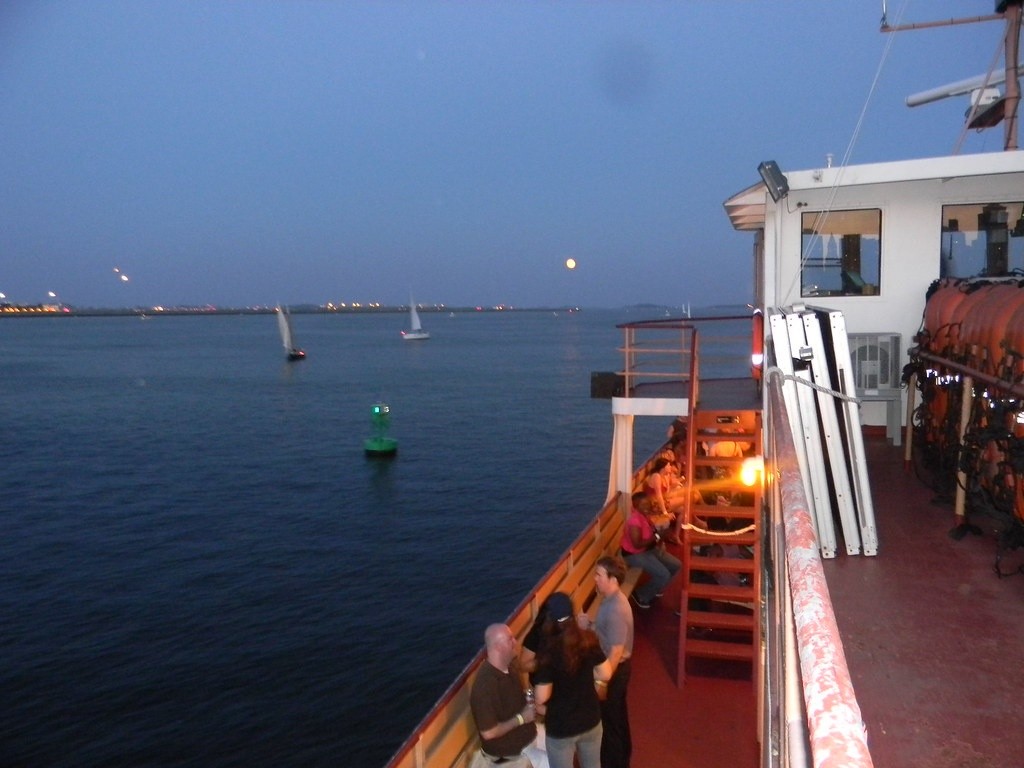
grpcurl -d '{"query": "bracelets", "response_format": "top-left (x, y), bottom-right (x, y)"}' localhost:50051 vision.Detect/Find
top-left (515, 712), bottom-right (525, 725)
top-left (595, 680), bottom-right (607, 687)
top-left (655, 533), bottom-right (660, 539)
top-left (664, 512), bottom-right (667, 515)
top-left (587, 620), bottom-right (592, 630)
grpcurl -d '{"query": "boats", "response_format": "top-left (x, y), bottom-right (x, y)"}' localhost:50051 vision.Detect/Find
top-left (364, 400), bottom-right (398, 455)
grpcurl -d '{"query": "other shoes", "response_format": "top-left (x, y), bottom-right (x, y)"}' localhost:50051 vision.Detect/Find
top-left (656, 591), bottom-right (664, 597)
top-left (631, 591), bottom-right (651, 609)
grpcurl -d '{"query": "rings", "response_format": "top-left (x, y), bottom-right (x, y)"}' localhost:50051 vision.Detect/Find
top-left (673, 519), bottom-right (674, 520)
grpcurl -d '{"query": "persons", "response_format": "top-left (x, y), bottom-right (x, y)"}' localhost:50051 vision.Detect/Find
top-left (469, 622), bottom-right (552, 768)
top-left (532, 592), bottom-right (614, 768)
top-left (575, 555), bottom-right (634, 768)
top-left (620, 416), bottom-right (755, 615)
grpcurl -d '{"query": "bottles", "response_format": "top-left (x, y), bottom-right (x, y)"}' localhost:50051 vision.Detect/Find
top-left (525, 689), bottom-right (535, 708)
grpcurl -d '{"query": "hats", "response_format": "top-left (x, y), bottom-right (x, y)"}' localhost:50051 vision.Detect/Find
top-left (546, 592), bottom-right (572, 623)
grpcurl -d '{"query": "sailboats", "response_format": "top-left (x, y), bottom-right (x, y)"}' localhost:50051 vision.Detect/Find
top-left (399, 292), bottom-right (431, 339)
top-left (275, 303), bottom-right (306, 359)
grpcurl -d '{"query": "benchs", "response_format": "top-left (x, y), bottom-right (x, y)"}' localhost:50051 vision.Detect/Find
top-left (386, 467), bottom-right (671, 768)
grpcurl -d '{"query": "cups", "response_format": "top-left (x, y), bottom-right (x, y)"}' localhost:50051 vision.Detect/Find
top-left (580, 615), bottom-right (588, 630)
